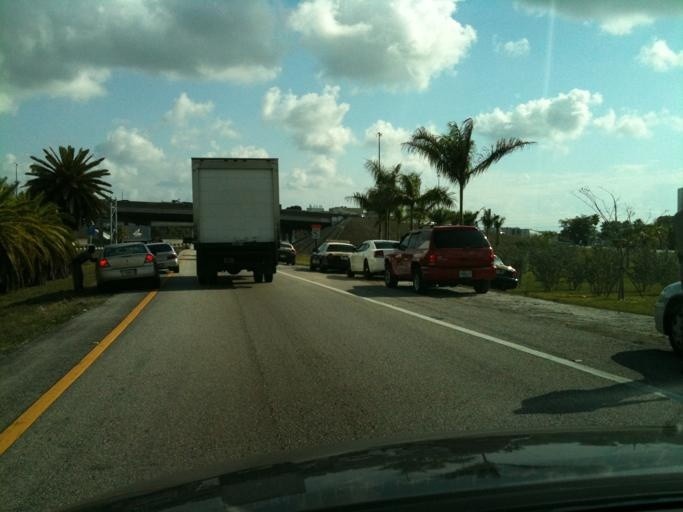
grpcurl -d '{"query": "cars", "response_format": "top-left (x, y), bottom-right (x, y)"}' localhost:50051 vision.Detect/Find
top-left (310, 241), bottom-right (356, 271)
top-left (279, 241), bottom-right (297, 263)
top-left (346, 241), bottom-right (400, 283)
top-left (286, 204), bottom-right (301, 212)
top-left (494, 253), bottom-right (518, 290)
top-left (142, 242), bottom-right (179, 273)
top-left (96, 243), bottom-right (163, 294)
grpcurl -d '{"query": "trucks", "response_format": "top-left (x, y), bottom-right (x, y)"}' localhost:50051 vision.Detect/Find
top-left (191, 157), bottom-right (279, 284)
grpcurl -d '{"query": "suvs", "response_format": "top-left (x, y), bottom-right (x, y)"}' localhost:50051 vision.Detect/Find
top-left (386, 227), bottom-right (494, 293)
top-left (656, 283), bottom-right (683, 354)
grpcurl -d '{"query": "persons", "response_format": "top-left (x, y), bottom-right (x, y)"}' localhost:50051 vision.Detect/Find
top-left (71, 245), bottom-right (97, 293)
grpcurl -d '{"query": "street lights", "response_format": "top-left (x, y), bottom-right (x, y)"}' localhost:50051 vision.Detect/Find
top-left (14, 162), bottom-right (19, 203)
top-left (377, 132), bottom-right (384, 239)
